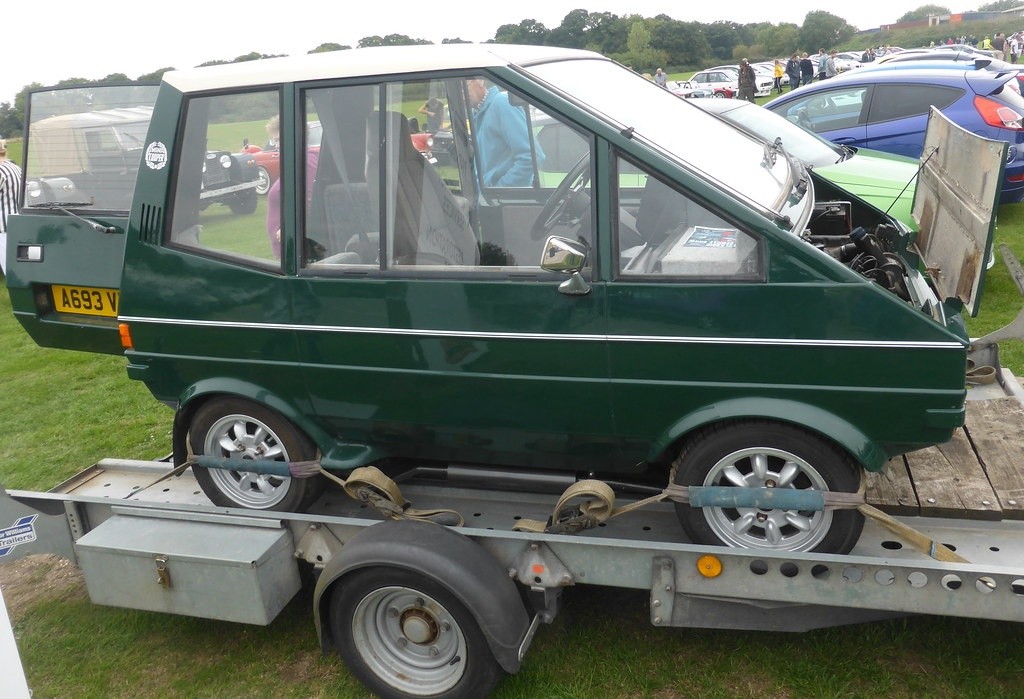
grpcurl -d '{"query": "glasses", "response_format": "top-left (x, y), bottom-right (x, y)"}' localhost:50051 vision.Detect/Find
top-left (270, 137), bottom-right (279, 146)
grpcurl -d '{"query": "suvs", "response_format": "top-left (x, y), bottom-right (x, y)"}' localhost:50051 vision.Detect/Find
top-left (30, 107), bottom-right (272, 225)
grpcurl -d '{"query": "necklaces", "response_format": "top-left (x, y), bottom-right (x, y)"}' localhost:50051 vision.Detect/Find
top-left (474, 91), bottom-right (489, 112)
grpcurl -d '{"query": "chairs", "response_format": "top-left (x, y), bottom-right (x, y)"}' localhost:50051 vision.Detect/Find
top-left (365, 110), bottom-right (480, 266)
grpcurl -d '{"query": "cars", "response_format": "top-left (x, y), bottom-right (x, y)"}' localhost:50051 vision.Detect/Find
top-left (682, 42), bottom-right (1024, 95)
top-left (3, 42), bottom-right (1012, 555)
top-left (761, 73), bottom-right (1024, 212)
top-left (239, 120), bottom-right (437, 194)
top-left (677, 68), bottom-right (771, 98)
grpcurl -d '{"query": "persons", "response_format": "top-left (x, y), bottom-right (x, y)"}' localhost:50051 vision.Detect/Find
top-left (982, 31), bottom-right (1024, 60)
top-left (785, 53), bottom-right (801, 90)
top-left (267, 114), bottom-right (320, 258)
top-left (0, 139), bottom-right (22, 275)
top-left (738, 58), bottom-right (759, 104)
top-left (826, 50), bottom-right (839, 78)
top-left (655, 68), bottom-right (668, 88)
top-left (930, 34), bottom-right (978, 47)
top-left (1010, 35), bottom-right (1021, 64)
top-left (862, 47), bottom-right (876, 63)
top-left (419, 97), bottom-right (444, 131)
top-left (881, 47), bottom-right (892, 57)
top-left (774, 60), bottom-right (783, 93)
top-left (461, 79), bottom-right (544, 186)
top-left (818, 48), bottom-right (828, 79)
top-left (989, 32), bottom-right (1005, 60)
top-left (800, 53), bottom-right (814, 85)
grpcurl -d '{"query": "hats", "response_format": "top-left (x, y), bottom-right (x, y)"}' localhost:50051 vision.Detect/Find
top-left (996, 32), bottom-right (1001, 36)
